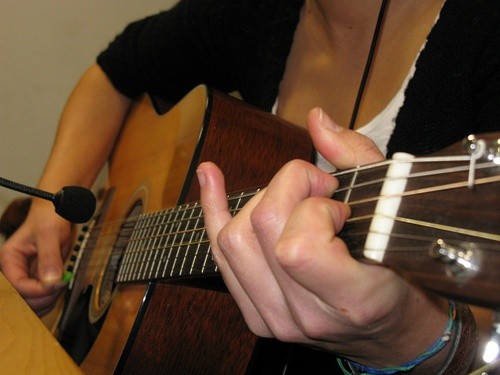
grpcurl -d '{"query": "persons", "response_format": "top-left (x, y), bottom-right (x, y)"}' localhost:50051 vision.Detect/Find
top-left (1, 0), bottom-right (500, 374)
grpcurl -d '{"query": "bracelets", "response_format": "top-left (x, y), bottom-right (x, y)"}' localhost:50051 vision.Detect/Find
top-left (337, 300), bottom-right (478, 375)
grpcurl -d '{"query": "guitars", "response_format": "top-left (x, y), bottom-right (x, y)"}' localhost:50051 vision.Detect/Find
top-left (38, 84), bottom-right (499, 374)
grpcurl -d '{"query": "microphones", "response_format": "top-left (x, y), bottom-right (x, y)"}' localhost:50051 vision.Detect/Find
top-left (0, 177), bottom-right (98, 226)
